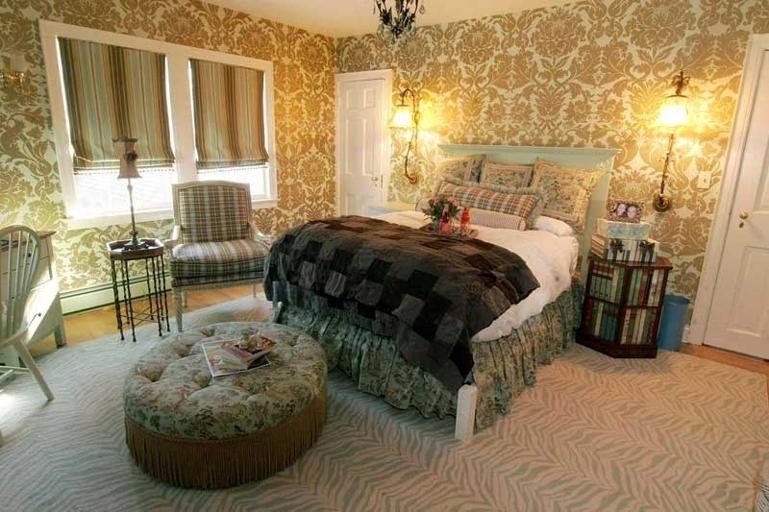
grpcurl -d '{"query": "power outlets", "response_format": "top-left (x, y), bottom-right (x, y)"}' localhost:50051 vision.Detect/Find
top-left (696, 171), bottom-right (712, 189)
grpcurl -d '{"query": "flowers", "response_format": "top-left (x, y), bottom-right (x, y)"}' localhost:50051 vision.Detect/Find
top-left (420, 194), bottom-right (470, 239)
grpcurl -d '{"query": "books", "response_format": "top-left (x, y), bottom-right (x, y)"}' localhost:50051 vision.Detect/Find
top-left (201, 332), bottom-right (278, 378)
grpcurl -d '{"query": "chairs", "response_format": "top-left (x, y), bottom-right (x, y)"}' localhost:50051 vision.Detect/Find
top-left (0, 224), bottom-right (55, 449)
top-left (164, 180), bottom-right (278, 334)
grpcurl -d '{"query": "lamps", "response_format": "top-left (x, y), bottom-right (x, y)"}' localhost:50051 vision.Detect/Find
top-left (650, 69), bottom-right (690, 212)
top-left (388, 89), bottom-right (419, 185)
top-left (0, 53), bottom-right (30, 95)
top-left (372, 0), bottom-right (426, 55)
top-left (112, 135), bottom-right (150, 250)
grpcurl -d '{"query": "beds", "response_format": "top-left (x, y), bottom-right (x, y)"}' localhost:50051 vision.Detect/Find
top-left (262, 144), bottom-right (622, 445)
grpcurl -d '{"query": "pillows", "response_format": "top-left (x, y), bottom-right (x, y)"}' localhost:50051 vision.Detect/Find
top-left (430, 174), bottom-right (549, 231)
top-left (479, 158), bottom-right (535, 188)
top-left (432, 153), bottom-right (487, 195)
top-left (414, 197), bottom-right (526, 231)
top-left (528, 156), bottom-right (615, 233)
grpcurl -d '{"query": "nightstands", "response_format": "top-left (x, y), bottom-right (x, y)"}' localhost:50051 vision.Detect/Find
top-left (574, 247), bottom-right (673, 359)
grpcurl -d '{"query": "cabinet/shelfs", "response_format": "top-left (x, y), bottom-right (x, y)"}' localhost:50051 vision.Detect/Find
top-left (0, 230), bottom-right (67, 380)
top-left (107, 237), bottom-right (171, 342)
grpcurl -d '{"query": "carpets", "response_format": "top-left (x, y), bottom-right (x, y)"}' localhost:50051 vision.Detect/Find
top-left (0, 291), bottom-right (769, 512)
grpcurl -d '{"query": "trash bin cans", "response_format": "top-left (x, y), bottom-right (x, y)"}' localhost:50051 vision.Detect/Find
top-left (656, 294), bottom-right (690, 352)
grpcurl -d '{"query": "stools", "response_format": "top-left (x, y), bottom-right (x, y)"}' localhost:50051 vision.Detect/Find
top-left (123, 321), bottom-right (329, 490)
